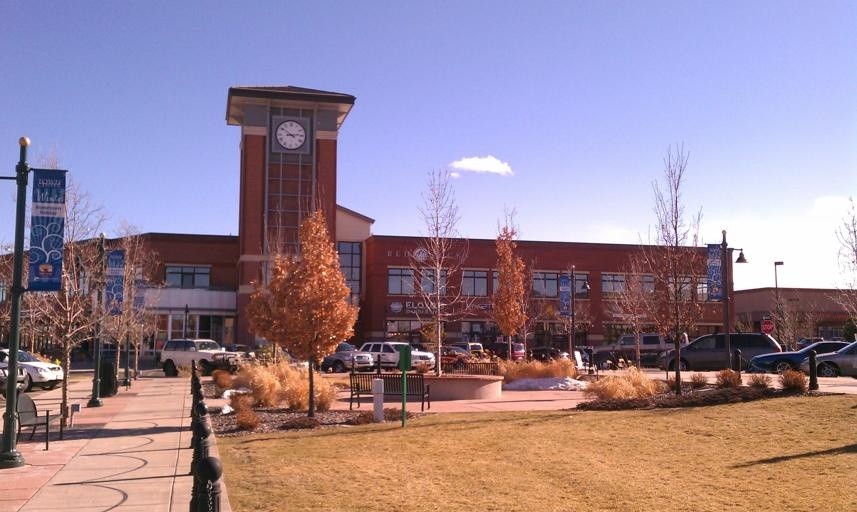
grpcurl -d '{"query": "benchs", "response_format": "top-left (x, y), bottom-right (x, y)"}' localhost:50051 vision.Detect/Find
top-left (348, 373), bottom-right (430, 412)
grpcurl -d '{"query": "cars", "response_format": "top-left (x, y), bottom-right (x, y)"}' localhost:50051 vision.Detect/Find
top-left (0, 348), bottom-right (64, 391)
top-left (746, 336), bottom-right (857, 378)
top-left (310, 339), bottom-right (596, 374)
top-left (0, 361), bottom-right (27, 399)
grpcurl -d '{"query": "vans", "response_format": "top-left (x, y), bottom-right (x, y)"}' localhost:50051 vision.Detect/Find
top-left (656, 330), bottom-right (783, 373)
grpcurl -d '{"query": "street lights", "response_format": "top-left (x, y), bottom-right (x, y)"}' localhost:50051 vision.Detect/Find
top-left (557, 263), bottom-right (592, 356)
top-left (184, 303), bottom-right (189, 339)
top-left (775, 261), bottom-right (784, 340)
top-left (702, 228), bottom-right (749, 369)
top-left (74, 232), bottom-right (126, 407)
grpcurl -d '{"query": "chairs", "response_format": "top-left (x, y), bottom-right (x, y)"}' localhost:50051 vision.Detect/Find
top-left (128, 369), bottom-right (137, 380)
top-left (118, 372), bottom-right (131, 391)
top-left (16, 392), bottom-right (63, 450)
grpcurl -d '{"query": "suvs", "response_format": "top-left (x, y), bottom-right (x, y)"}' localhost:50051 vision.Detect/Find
top-left (160, 338), bottom-right (256, 377)
top-left (594, 332), bottom-right (690, 370)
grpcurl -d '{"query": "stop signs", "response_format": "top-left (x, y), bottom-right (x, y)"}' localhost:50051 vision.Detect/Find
top-left (761, 321), bottom-right (775, 333)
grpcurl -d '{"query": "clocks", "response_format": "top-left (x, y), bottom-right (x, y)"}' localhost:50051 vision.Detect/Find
top-left (270, 115), bottom-right (311, 154)
